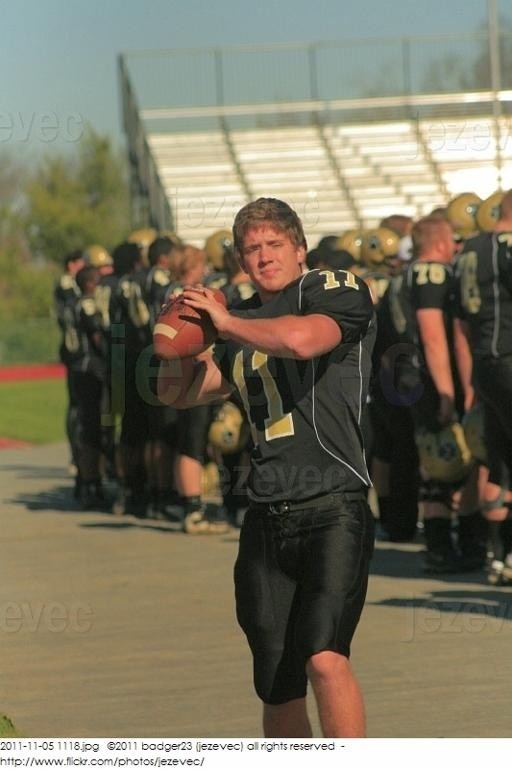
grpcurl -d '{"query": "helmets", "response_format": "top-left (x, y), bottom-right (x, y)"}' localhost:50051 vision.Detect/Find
top-left (82, 229), bottom-right (180, 268)
top-left (415, 421), bottom-right (473, 486)
top-left (205, 230), bottom-right (234, 270)
top-left (204, 400), bottom-right (249, 454)
top-left (334, 192), bottom-right (508, 271)
top-left (462, 400), bottom-right (510, 468)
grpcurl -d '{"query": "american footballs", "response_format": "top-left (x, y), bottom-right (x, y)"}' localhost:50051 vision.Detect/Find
top-left (153, 287), bottom-right (227, 359)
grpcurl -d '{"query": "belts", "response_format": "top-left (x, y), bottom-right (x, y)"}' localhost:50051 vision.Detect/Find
top-left (248, 493), bottom-right (362, 515)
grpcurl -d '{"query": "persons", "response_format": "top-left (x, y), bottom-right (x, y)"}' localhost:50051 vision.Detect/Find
top-left (57, 188), bottom-right (512, 738)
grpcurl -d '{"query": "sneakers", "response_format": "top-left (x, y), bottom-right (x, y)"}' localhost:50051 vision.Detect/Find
top-left (183, 510), bottom-right (231, 535)
